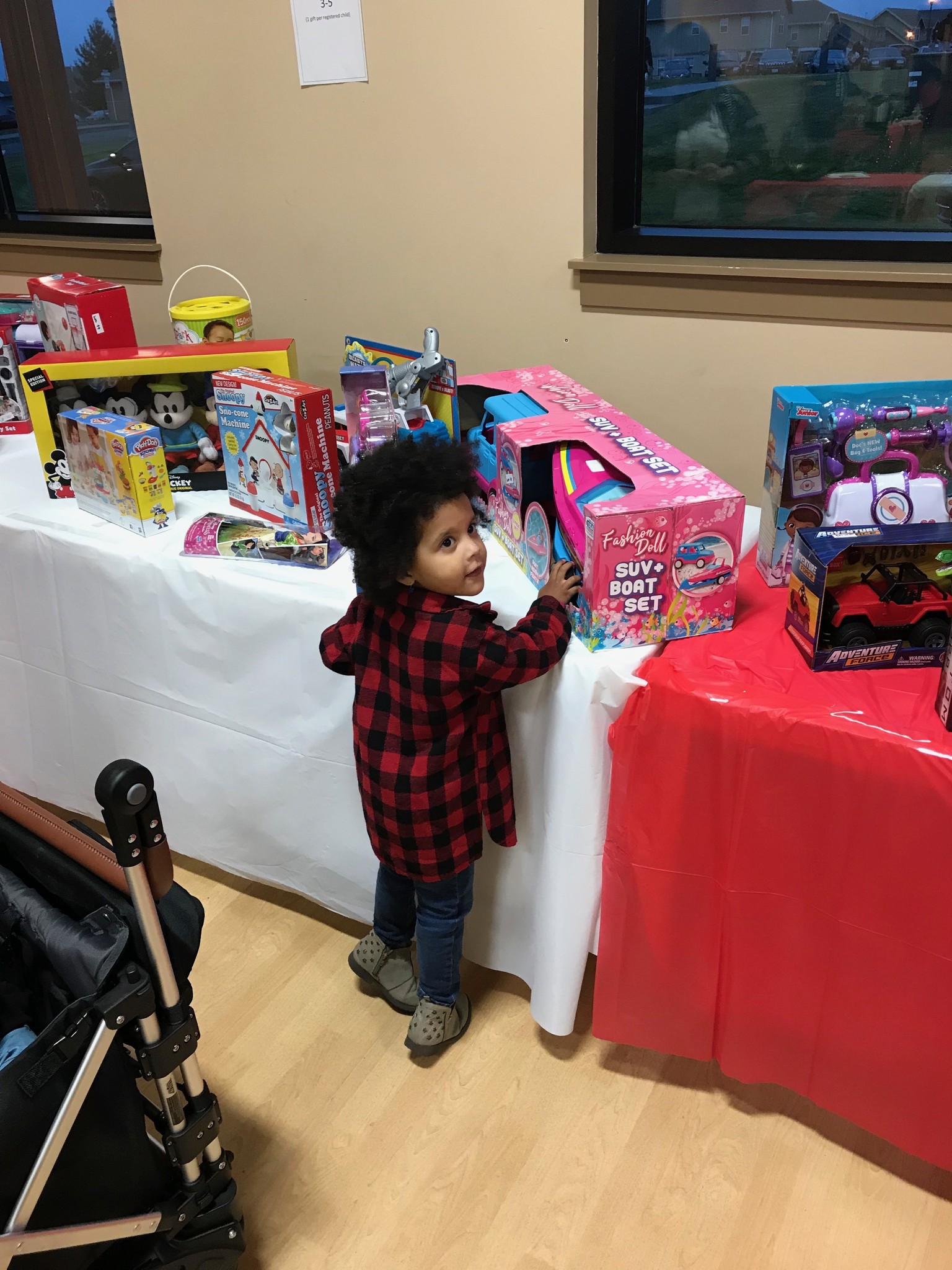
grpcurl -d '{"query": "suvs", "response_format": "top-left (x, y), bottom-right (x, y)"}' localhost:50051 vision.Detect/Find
top-left (823, 561), bottom-right (952, 651)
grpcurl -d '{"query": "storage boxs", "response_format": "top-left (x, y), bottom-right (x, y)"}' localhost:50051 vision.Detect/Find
top-left (211, 365), bottom-right (341, 534)
top-left (18, 338), bottom-right (300, 499)
top-left (755, 380), bottom-right (952, 589)
top-left (0, 292), bottom-right (38, 325)
top-left (57, 405), bottom-right (178, 538)
top-left (340, 364), bottom-right (398, 459)
top-left (457, 365), bottom-right (746, 654)
top-left (0, 325), bottom-right (34, 435)
top-left (784, 521), bottom-right (952, 673)
top-left (27, 271), bottom-right (137, 353)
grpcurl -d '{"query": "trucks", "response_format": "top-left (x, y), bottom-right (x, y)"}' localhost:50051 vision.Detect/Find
top-left (467, 392), bottom-right (635, 610)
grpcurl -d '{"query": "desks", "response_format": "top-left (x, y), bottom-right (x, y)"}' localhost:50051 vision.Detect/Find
top-left (590, 541), bottom-right (952, 1173)
top-left (743, 172), bottom-right (924, 225)
top-left (827, 124), bottom-right (905, 162)
top-left (0, 429), bottom-right (761, 1036)
top-left (903, 172), bottom-right (952, 229)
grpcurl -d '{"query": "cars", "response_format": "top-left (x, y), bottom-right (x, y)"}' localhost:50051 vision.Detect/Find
top-left (85, 136), bottom-right (147, 216)
top-left (660, 42), bottom-right (952, 82)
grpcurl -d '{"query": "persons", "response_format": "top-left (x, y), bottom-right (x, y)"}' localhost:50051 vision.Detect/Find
top-left (63, 418), bottom-right (112, 482)
top-left (202, 320), bottom-right (235, 344)
top-left (319, 430), bottom-right (582, 1055)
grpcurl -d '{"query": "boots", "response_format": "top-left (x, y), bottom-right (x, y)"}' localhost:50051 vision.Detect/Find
top-left (404, 992), bottom-right (471, 1056)
top-left (349, 928), bottom-right (418, 1011)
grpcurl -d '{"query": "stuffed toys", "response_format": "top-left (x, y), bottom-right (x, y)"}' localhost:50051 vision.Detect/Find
top-left (50, 371), bottom-right (226, 475)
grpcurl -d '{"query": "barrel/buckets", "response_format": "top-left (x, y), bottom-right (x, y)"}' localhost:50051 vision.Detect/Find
top-left (168, 265), bottom-right (256, 345)
